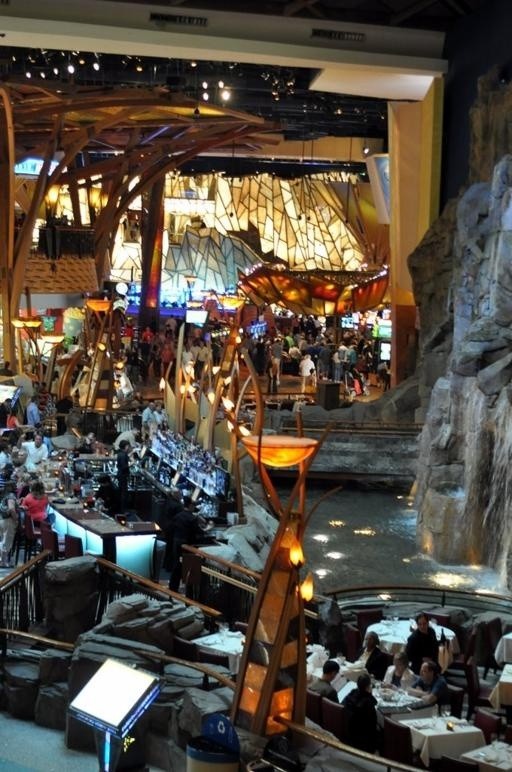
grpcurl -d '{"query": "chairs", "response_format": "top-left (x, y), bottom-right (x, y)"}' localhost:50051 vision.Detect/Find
top-left (267, 594), bottom-right (510, 767)
top-left (1, 430), bottom-right (275, 612)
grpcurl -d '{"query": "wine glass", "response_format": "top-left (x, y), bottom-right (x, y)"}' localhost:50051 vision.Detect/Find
top-left (219, 623), bottom-right (230, 644)
top-left (440, 704), bottom-right (452, 729)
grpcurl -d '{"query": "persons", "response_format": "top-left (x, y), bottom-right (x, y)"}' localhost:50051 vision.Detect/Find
top-left (227, 301), bottom-right (392, 401)
top-left (381, 651), bottom-right (415, 694)
top-left (307, 661), bottom-right (340, 704)
top-left (342, 674), bottom-right (384, 741)
top-left (353, 631), bottom-right (384, 681)
top-left (404, 614), bottom-right (440, 675)
top-left (408, 659), bottom-right (453, 715)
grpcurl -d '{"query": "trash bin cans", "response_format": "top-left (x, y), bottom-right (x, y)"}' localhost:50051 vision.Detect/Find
top-left (186, 714), bottom-right (303, 772)
top-left (317, 380), bottom-right (341, 411)
